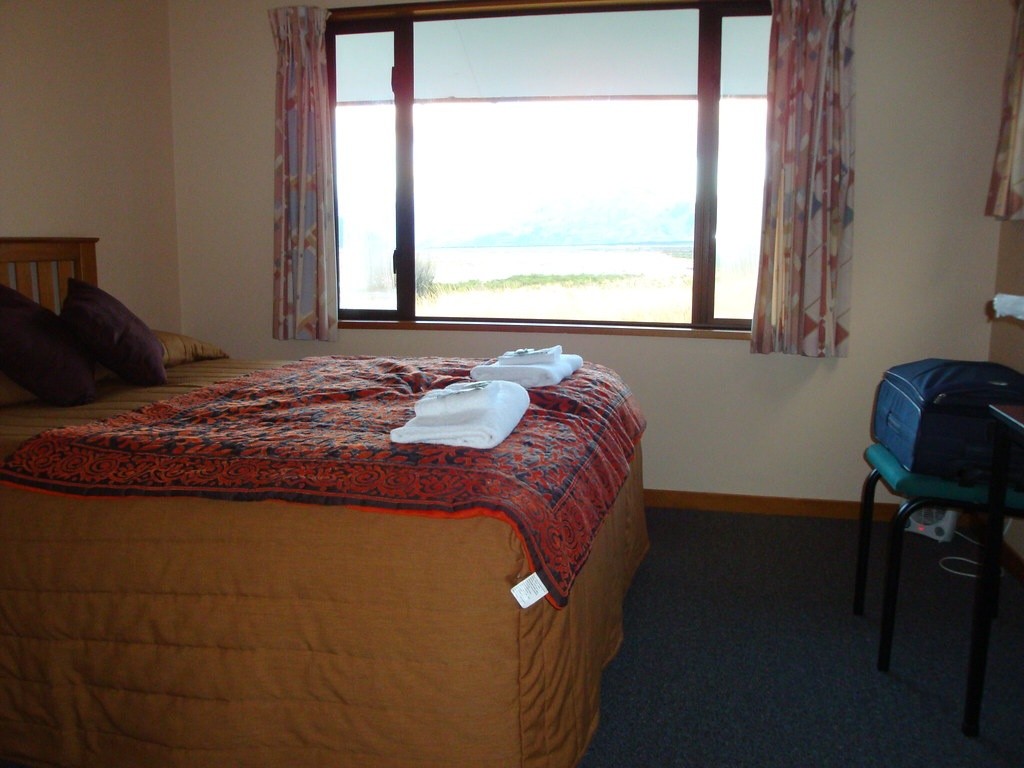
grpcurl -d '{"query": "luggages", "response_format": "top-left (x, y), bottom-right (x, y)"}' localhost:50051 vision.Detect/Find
top-left (869, 357), bottom-right (1024, 477)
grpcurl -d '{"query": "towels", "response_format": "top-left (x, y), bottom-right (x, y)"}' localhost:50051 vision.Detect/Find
top-left (389, 380), bottom-right (531, 450)
top-left (470, 344), bottom-right (584, 386)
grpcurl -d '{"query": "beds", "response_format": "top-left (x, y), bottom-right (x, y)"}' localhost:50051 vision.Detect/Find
top-left (0, 236), bottom-right (649, 767)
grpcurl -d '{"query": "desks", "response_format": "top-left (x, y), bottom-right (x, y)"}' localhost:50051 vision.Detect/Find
top-left (962, 403), bottom-right (1024, 736)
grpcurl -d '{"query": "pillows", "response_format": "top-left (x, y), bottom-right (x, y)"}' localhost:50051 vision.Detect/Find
top-left (0, 282), bottom-right (96, 405)
top-left (60, 277), bottom-right (167, 387)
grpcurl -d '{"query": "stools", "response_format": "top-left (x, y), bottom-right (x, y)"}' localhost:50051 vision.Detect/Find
top-left (853, 445), bottom-right (1024, 673)
